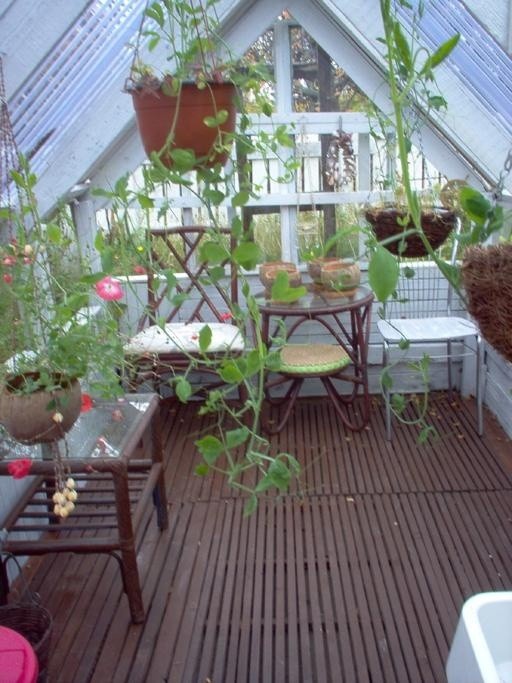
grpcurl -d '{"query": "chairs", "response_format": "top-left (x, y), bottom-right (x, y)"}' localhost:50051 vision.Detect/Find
top-left (118, 223), bottom-right (256, 449)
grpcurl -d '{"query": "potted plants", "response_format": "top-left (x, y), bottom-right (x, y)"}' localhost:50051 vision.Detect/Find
top-left (92, 0), bottom-right (309, 520)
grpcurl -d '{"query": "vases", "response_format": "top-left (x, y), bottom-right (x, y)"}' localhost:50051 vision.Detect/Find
top-left (258, 256), bottom-right (360, 304)
top-left (0, 370), bottom-right (85, 446)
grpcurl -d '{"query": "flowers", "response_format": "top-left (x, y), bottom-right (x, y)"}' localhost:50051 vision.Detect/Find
top-left (0, 147), bottom-right (175, 479)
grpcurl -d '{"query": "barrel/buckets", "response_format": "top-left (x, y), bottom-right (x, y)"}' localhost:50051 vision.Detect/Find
top-left (0, 551), bottom-right (54, 683)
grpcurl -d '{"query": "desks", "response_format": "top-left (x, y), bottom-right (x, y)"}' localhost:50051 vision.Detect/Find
top-left (0, 390), bottom-right (171, 623)
top-left (246, 285), bottom-right (376, 434)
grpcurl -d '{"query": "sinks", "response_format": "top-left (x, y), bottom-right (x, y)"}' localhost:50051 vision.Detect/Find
top-left (445, 591), bottom-right (512, 683)
